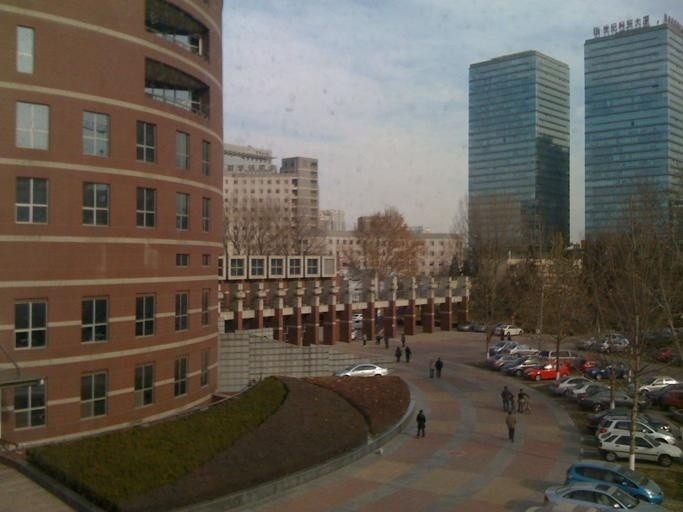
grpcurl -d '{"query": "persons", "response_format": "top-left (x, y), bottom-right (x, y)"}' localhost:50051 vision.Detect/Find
top-left (361, 332), bottom-right (366, 347)
top-left (400, 334), bottom-right (405, 348)
top-left (505, 411), bottom-right (516, 443)
top-left (428, 359), bottom-right (435, 378)
top-left (516, 389), bottom-right (529, 413)
top-left (404, 346), bottom-right (410, 363)
top-left (415, 410), bottom-right (425, 437)
top-left (435, 357), bottom-right (442, 378)
top-left (394, 346), bottom-right (401, 362)
top-left (500, 386), bottom-right (513, 412)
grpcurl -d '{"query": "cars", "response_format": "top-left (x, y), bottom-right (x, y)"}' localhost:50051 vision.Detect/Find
top-left (351, 313), bottom-right (362, 321)
top-left (485, 331), bottom-right (682, 512)
top-left (335, 362), bottom-right (389, 379)
top-left (456, 320), bottom-right (525, 337)
